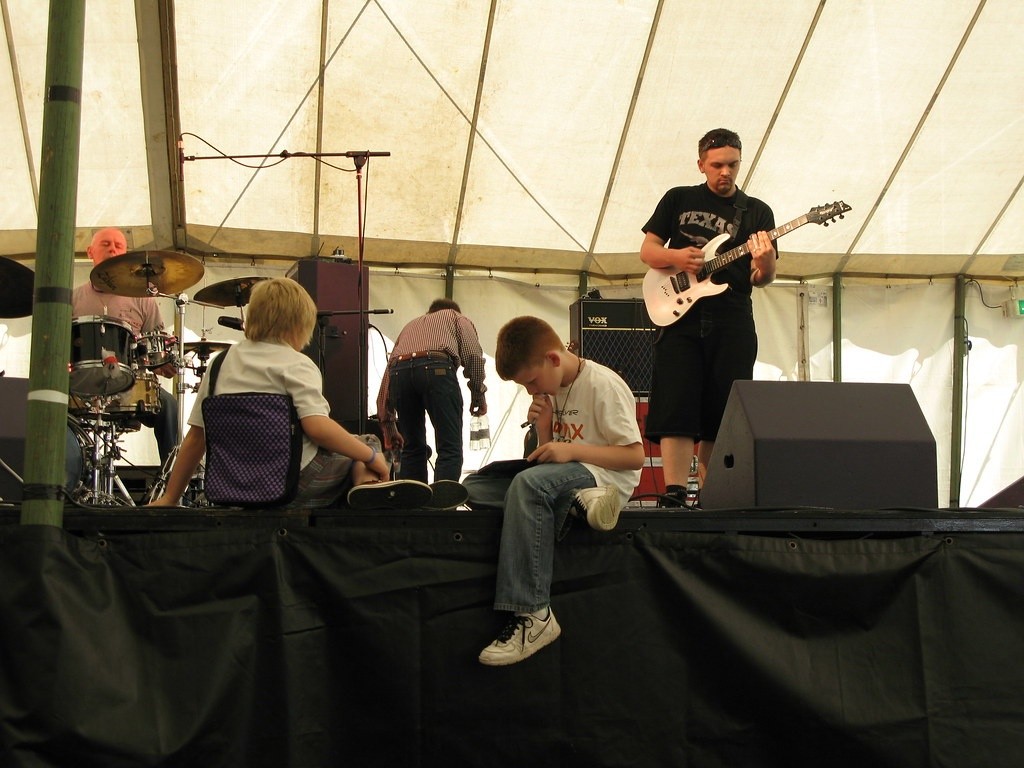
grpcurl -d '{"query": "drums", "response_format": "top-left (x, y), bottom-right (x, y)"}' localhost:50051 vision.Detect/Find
top-left (68, 314), bottom-right (136, 396)
top-left (69, 374), bottom-right (161, 414)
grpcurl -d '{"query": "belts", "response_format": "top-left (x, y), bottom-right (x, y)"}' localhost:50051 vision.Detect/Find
top-left (387, 351), bottom-right (454, 367)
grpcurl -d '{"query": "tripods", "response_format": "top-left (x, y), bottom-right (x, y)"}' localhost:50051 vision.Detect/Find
top-left (72, 395), bottom-right (138, 510)
top-left (147, 281), bottom-right (225, 508)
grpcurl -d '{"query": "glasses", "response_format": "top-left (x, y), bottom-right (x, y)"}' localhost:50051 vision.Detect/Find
top-left (700, 137), bottom-right (742, 158)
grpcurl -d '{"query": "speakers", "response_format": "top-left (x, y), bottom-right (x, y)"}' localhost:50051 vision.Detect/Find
top-left (0, 377), bottom-right (30, 504)
top-left (569, 298), bottom-right (663, 397)
top-left (296, 258), bottom-right (370, 436)
top-left (699, 380), bottom-right (939, 513)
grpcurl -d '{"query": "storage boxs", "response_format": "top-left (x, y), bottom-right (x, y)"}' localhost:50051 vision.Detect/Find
top-left (626, 397), bottom-right (699, 506)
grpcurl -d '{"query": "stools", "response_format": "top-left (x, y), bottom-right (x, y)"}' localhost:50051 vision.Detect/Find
top-left (67, 417), bottom-right (143, 505)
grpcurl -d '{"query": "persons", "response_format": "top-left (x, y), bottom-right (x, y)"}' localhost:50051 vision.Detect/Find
top-left (377, 298), bottom-right (487, 485)
top-left (143, 277), bottom-right (469, 507)
top-left (462, 316), bottom-right (645, 665)
top-left (71, 228), bottom-right (191, 508)
top-left (641, 128), bottom-right (779, 508)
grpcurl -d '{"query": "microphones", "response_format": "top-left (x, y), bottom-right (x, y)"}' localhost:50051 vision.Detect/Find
top-left (219, 317), bottom-right (246, 331)
top-left (177, 136), bottom-right (184, 181)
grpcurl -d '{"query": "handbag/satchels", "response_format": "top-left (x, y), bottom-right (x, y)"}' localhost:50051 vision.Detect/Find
top-left (201, 346), bottom-right (304, 507)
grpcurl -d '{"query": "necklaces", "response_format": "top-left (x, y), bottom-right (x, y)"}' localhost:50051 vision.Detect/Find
top-left (553, 356), bottom-right (583, 442)
top-left (90, 281), bottom-right (113, 316)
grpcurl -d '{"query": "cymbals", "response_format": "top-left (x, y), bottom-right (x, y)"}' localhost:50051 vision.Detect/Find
top-left (90, 251), bottom-right (204, 298)
top-left (183, 341), bottom-right (232, 355)
top-left (194, 277), bottom-right (271, 308)
top-left (0, 255), bottom-right (37, 320)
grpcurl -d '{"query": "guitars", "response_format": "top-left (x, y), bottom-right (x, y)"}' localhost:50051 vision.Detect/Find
top-left (642, 199), bottom-right (852, 326)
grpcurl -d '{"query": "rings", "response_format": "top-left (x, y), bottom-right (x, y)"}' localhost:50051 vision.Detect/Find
top-left (754, 247), bottom-right (760, 253)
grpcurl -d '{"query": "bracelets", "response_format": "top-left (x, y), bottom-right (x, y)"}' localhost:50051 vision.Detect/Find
top-left (362, 443), bottom-right (375, 465)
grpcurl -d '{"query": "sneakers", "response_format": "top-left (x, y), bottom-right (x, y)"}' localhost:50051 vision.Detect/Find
top-left (575, 484), bottom-right (621, 531)
top-left (477, 605), bottom-right (561, 666)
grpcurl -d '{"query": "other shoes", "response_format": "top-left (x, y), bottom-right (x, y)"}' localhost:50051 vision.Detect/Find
top-left (346, 480), bottom-right (471, 512)
top-left (660, 485), bottom-right (687, 507)
